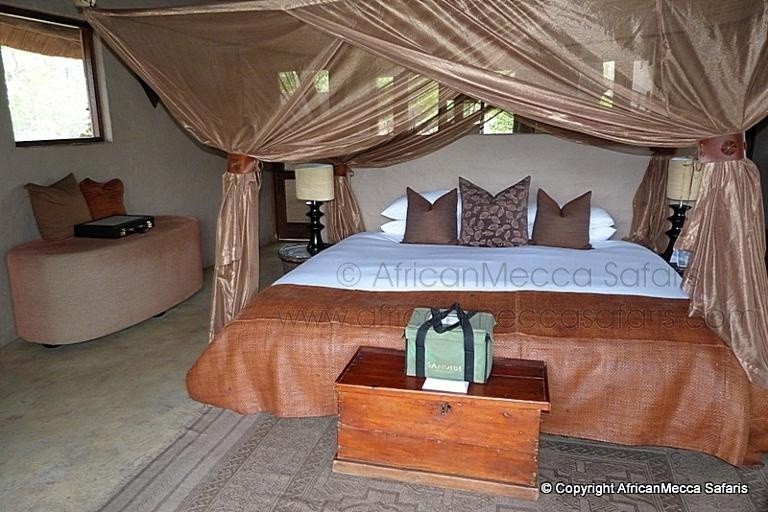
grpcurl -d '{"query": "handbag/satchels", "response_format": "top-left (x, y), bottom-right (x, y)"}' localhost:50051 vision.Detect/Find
top-left (400, 302), bottom-right (498, 384)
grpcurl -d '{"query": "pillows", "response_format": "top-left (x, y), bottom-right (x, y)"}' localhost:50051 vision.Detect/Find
top-left (77, 179), bottom-right (130, 218)
top-left (377, 176), bottom-right (618, 257)
top-left (23, 172), bottom-right (93, 241)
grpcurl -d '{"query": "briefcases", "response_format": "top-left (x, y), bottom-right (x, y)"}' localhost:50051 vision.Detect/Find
top-left (75, 214), bottom-right (155, 239)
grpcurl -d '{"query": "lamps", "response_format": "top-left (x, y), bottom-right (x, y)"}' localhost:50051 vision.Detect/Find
top-left (657, 156), bottom-right (702, 267)
top-left (292, 160), bottom-right (338, 253)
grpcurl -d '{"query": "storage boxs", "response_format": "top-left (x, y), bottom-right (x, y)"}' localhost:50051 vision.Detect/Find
top-left (326, 343), bottom-right (551, 502)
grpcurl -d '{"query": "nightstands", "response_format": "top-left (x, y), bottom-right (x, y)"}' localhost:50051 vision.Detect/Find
top-left (278, 243), bottom-right (314, 275)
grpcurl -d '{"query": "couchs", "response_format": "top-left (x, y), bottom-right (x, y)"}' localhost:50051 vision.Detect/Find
top-left (7, 214), bottom-right (206, 350)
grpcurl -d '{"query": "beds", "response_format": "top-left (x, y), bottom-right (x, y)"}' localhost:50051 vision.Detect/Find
top-left (183, 124), bottom-right (767, 470)
